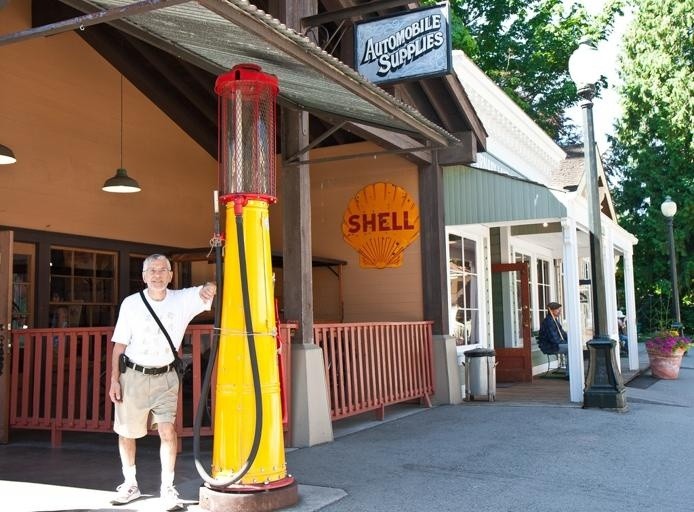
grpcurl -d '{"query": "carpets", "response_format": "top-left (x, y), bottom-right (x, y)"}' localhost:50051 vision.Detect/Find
top-left (625, 372), bottom-right (660, 392)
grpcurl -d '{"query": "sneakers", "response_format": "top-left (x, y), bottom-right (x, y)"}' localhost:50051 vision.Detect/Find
top-left (160, 485), bottom-right (184, 511)
top-left (111, 481), bottom-right (141, 505)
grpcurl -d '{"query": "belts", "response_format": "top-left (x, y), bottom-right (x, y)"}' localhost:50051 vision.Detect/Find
top-left (127, 362), bottom-right (174, 376)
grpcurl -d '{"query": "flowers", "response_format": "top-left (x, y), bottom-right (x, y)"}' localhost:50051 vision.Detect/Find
top-left (644, 326), bottom-right (692, 358)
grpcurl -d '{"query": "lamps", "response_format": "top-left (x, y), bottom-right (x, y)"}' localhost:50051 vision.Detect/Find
top-left (0, 142), bottom-right (18, 168)
top-left (98, 69), bottom-right (145, 197)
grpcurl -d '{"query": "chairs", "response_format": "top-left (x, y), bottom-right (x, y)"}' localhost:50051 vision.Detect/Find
top-left (531, 326), bottom-right (569, 378)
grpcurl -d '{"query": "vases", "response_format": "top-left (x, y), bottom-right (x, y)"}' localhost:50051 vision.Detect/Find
top-left (647, 346), bottom-right (685, 381)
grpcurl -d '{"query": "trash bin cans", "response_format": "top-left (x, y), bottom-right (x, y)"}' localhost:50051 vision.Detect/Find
top-left (461, 348), bottom-right (499, 402)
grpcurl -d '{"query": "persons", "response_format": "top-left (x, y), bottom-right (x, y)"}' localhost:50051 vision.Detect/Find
top-left (110, 253), bottom-right (220, 511)
top-left (617, 311), bottom-right (628, 356)
top-left (51, 293), bottom-right (70, 350)
top-left (540, 302), bottom-right (570, 381)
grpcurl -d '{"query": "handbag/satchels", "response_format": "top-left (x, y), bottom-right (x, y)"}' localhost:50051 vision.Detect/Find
top-left (175, 355), bottom-right (188, 378)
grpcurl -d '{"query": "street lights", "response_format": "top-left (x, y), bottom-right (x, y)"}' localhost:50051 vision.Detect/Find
top-left (659, 192), bottom-right (690, 356)
top-left (564, 40), bottom-right (629, 412)
